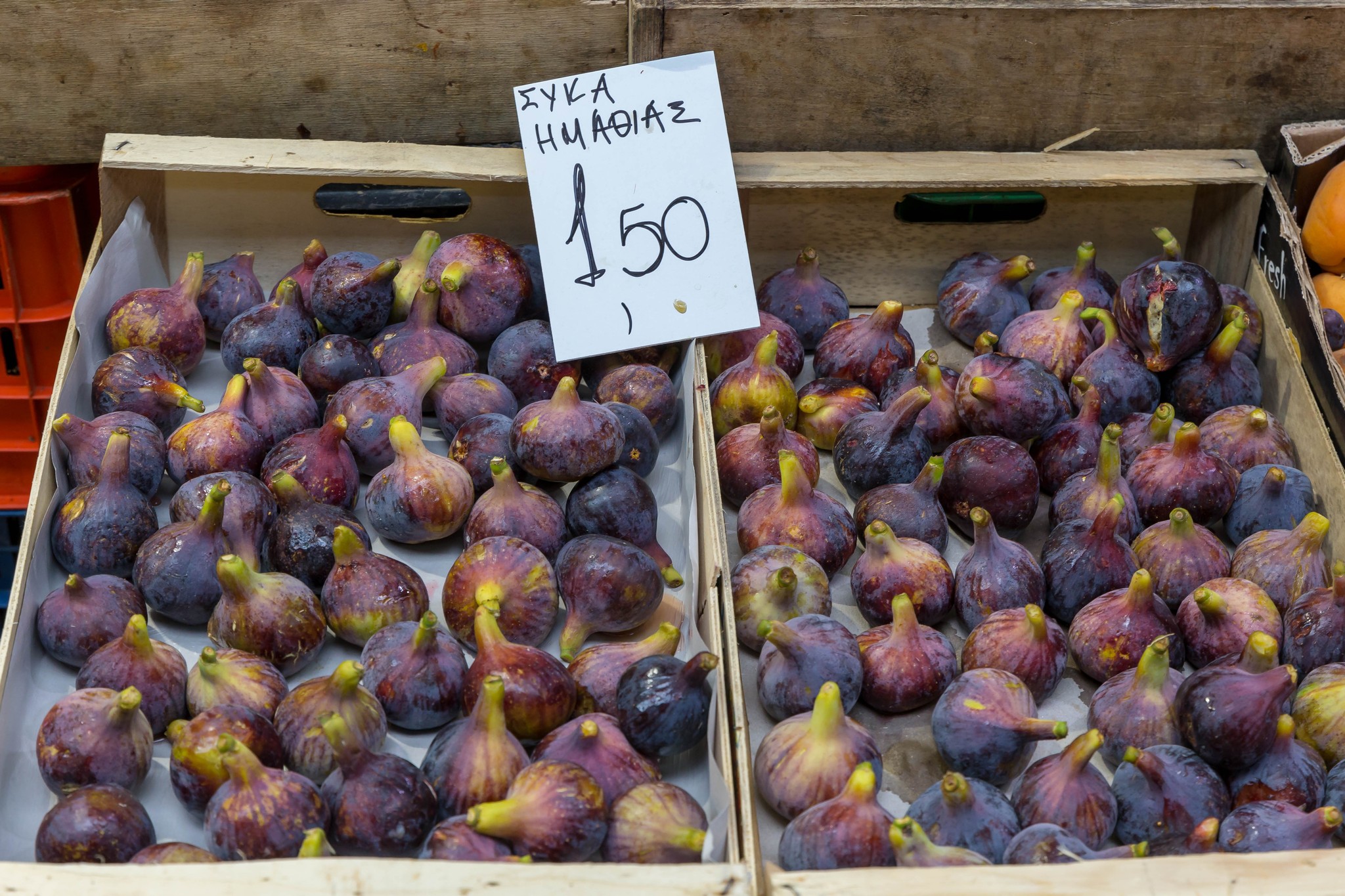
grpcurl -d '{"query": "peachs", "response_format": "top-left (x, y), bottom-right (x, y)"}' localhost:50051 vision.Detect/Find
top-left (1300, 157), bottom-right (1345, 381)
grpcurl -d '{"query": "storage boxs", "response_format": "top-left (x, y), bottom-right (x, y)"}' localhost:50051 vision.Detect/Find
top-left (700, 151), bottom-right (1345, 896)
top-left (0, 511), bottom-right (35, 614)
top-left (0, 133), bottom-right (746, 896)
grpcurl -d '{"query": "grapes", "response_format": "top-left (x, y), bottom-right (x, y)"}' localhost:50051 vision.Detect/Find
top-left (1321, 308), bottom-right (1345, 351)
